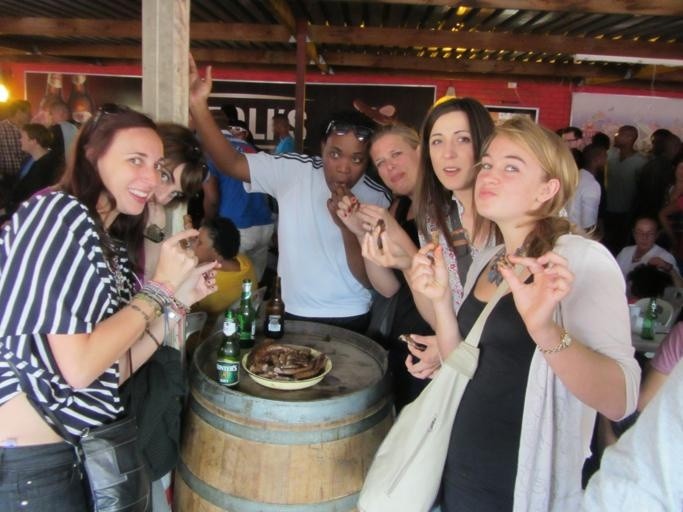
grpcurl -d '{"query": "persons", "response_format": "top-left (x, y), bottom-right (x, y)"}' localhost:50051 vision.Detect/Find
top-left (1, 52), bottom-right (683, 512)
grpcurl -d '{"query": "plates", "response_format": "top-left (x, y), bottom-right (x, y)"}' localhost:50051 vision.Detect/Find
top-left (241, 344), bottom-right (332, 390)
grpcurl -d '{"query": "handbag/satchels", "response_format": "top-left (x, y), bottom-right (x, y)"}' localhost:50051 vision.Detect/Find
top-left (356, 339), bottom-right (481, 512)
top-left (80, 409), bottom-right (151, 512)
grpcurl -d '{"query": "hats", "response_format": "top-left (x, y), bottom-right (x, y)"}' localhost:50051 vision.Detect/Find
top-left (210, 110), bottom-right (233, 131)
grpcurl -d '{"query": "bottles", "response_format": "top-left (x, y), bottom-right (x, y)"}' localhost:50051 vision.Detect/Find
top-left (236, 279), bottom-right (256, 348)
top-left (39, 70), bottom-right (65, 111)
top-left (66, 74), bottom-right (96, 128)
top-left (641, 297), bottom-right (657, 340)
top-left (215, 310), bottom-right (240, 389)
top-left (264, 277), bottom-right (284, 339)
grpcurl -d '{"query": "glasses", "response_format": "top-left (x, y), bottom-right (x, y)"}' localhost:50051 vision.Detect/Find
top-left (185, 142), bottom-right (210, 184)
top-left (91, 101), bottom-right (132, 133)
top-left (321, 120), bottom-right (375, 147)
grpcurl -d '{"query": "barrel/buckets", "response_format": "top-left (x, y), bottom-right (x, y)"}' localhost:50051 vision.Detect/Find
top-left (173, 317), bottom-right (397, 511)
